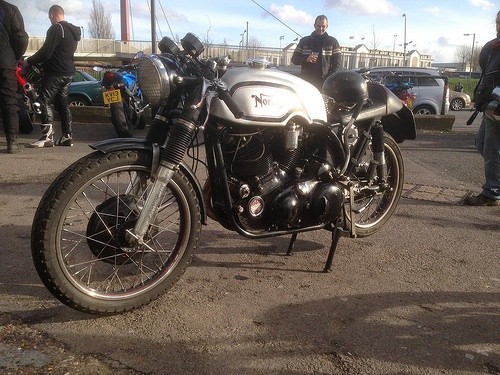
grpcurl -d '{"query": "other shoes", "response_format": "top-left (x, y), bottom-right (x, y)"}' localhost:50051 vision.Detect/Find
top-left (54, 133), bottom-right (74, 146)
top-left (7, 142), bottom-right (25, 154)
top-left (29, 133), bottom-right (55, 148)
top-left (465, 192), bottom-right (500, 206)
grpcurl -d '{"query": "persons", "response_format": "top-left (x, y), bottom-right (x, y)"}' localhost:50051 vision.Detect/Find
top-left (291, 15), bottom-right (341, 92)
top-left (454, 82), bottom-right (463, 93)
top-left (19, 5), bottom-right (81, 148)
top-left (465, 11), bottom-right (500, 205)
top-left (0, 0), bottom-right (29, 154)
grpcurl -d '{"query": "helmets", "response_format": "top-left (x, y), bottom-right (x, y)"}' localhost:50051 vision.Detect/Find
top-left (323, 69), bottom-right (369, 111)
top-left (21, 64), bottom-right (43, 83)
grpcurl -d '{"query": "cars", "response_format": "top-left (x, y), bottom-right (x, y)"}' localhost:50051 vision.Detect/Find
top-left (449, 90), bottom-right (471, 112)
top-left (352, 66), bottom-right (451, 116)
top-left (67, 69), bottom-right (107, 107)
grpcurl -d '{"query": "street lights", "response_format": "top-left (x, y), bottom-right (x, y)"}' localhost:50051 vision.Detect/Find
top-left (402, 14), bottom-right (408, 67)
top-left (463, 33), bottom-right (477, 81)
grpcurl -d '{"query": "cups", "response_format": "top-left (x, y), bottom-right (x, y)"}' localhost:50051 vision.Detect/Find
top-left (311, 52), bottom-right (318, 63)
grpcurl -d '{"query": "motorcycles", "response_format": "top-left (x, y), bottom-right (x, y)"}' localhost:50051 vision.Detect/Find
top-left (92, 50), bottom-right (148, 138)
top-left (29, 32), bottom-right (417, 316)
